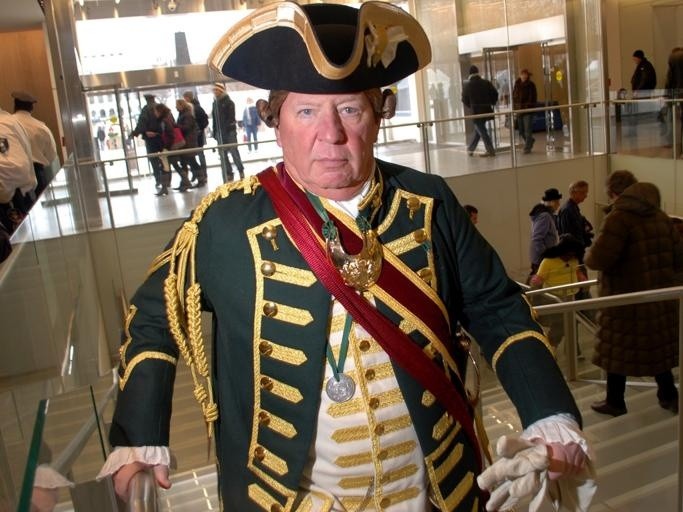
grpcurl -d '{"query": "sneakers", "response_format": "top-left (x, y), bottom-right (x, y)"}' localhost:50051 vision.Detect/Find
top-left (466, 147), bottom-right (496, 158)
top-left (225, 168), bottom-right (246, 180)
top-left (656, 395), bottom-right (678, 413)
top-left (590, 399), bottom-right (626, 416)
top-left (659, 129), bottom-right (673, 149)
top-left (521, 137), bottom-right (536, 155)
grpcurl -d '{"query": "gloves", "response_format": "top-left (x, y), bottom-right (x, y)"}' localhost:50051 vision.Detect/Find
top-left (478, 433), bottom-right (594, 510)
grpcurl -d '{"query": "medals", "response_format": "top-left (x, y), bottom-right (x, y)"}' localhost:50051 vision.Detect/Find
top-left (324, 372), bottom-right (355, 403)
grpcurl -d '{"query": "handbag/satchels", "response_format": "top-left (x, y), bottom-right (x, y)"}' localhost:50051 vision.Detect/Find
top-left (162, 128), bottom-right (186, 151)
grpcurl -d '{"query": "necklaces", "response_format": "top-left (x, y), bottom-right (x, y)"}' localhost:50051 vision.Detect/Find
top-left (300, 189), bottom-right (384, 291)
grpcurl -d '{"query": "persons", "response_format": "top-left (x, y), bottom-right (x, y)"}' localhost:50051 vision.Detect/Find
top-left (127, 81), bottom-right (263, 194)
top-left (93, 1), bottom-right (598, 511)
top-left (97, 126), bottom-right (105, 151)
top-left (464, 204), bottom-right (479, 228)
top-left (511, 69), bottom-right (536, 155)
top-left (497, 77), bottom-right (509, 105)
top-left (614, 86), bottom-right (627, 123)
top-left (622, 50), bottom-right (667, 139)
top-left (1, 91), bottom-right (58, 263)
top-left (661, 47), bottom-right (683, 148)
top-left (525, 168), bottom-right (680, 418)
top-left (461, 65), bottom-right (498, 158)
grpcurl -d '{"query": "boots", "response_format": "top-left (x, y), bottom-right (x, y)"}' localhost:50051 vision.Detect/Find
top-left (155, 167), bottom-right (208, 196)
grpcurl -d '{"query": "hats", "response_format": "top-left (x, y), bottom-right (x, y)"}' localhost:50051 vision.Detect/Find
top-left (12, 90), bottom-right (37, 106)
top-left (469, 65), bottom-right (479, 72)
top-left (205, 1), bottom-right (432, 95)
top-left (519, 69), bottom-right (533, 76)
top-left (540, 188), bottom-right (563, 202)
